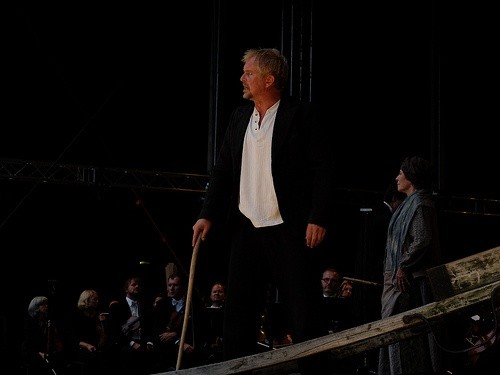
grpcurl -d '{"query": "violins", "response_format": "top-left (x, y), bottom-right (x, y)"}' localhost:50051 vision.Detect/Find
top-left (166, 305), bottom-right (185, 332)
top-left (121, 315), bottom-right (142, 336)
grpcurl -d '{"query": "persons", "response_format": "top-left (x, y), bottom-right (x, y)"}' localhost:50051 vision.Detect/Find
top-left (365, 192), bottom-right (406, 375)
top-left (21, 260), bottom-right (355, 375)
top-left (191, 47), bottom-right (334, 375)
top-left (377, 156), bottom-right (446, 375)
